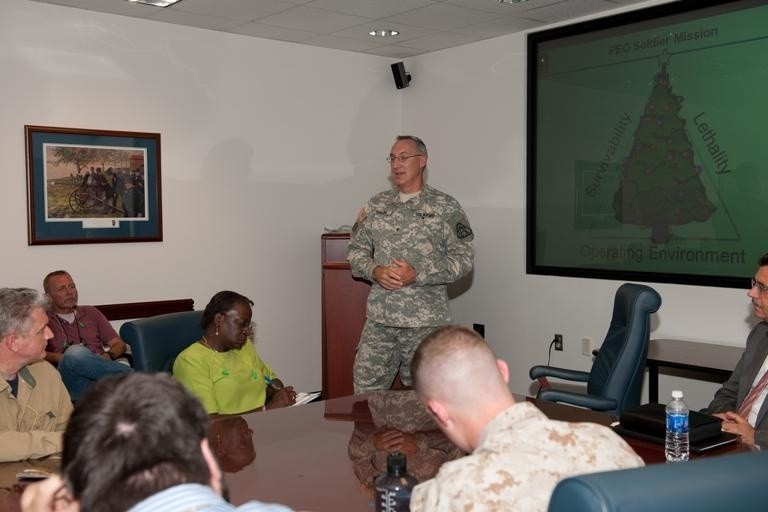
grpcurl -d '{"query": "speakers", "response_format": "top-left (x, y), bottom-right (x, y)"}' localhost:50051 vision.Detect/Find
top-left (390, 61), bottom-right (410, 90)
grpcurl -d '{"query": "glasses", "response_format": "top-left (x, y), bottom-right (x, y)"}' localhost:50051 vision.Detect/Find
top-left (750, 277), bottom-right (768, 293)
top-left (386, 155), bottom-right (422, 163)
top-left (221, 313), bottom-right (257, 331)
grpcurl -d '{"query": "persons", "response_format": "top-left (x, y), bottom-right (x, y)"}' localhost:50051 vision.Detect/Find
top-left (350, 397), bottom-right (464, 502)
top-left (409, 326), bottom-right (645, 511)
top-left (1, 289), bottom-right (73, 461)
top-left (19, 371), bottom-right (292, 512)
top-left (174, 290), bottom-right (297, 416)
top-left (44, 270), bottom-right (135, 403)
top-left (81, 167), bottom-right (144, 218)
top-left (346, 135), bottom-right (474, 395)
top-left (700, 261), bottom-right (768, 449)
top-left (204, 413), bottom-right (259, 472)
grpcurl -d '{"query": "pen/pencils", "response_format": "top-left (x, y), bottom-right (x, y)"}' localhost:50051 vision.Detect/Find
top-left (264, 376), bottom-right (281, 391)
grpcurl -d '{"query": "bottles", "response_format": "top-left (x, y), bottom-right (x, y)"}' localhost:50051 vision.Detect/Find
top-left (374, 452), bottom-right (419, 511)
top-left (665, 390), bottom-right (689, 463)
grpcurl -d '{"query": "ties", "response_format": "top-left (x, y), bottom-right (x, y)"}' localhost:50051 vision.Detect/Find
top-left (738, 372), bottom-right (768, 421)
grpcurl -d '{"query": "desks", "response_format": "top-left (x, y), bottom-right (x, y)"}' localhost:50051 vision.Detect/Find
top-left (0, 392), bottom-right (660, 512)
top-left (592, 339), bottom-right (745, 401)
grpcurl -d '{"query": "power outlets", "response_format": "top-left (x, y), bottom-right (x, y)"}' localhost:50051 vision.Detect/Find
top-left (554, 334), bottom-right (563, 351)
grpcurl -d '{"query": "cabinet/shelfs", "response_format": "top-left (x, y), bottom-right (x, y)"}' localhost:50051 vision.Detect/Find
top-left (319, 232), bottom-right (372, 398)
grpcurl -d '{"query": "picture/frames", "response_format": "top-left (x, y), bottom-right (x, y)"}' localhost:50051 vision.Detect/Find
top-left (25, 123), bottom-right (164, 243)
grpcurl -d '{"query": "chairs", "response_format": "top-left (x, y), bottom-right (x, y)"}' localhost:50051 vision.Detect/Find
top-left (547, 449), bottom-right (765, 512)
top-left (528, 283), bottom-right (661, 422)
top-left (120, 311), bottom-right (210, 374)
top-left (113, 354), bottom-right (134, 368)
